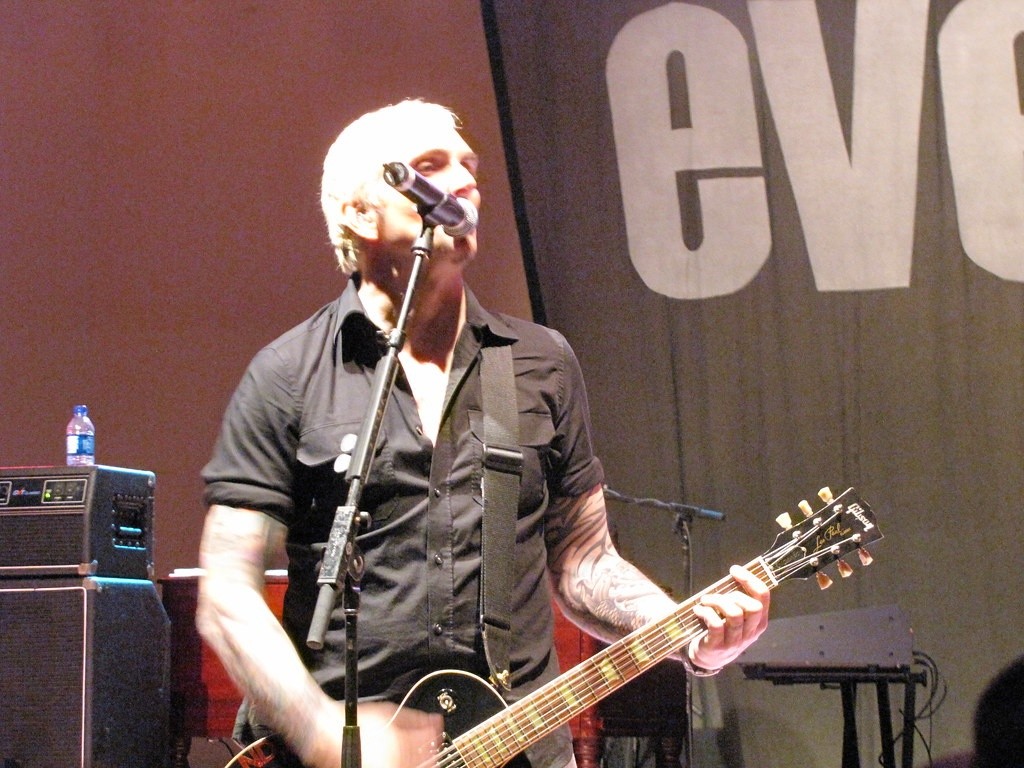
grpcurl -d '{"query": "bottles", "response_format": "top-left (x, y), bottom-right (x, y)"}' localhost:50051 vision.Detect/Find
top-left (66, 404), bottom-right (95, 467)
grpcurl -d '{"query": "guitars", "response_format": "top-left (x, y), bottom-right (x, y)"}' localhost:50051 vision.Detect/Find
top-left (225, 480), bottom-right (883, 768)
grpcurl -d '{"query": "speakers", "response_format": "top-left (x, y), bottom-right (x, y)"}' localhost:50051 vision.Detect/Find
top-left (0, 574), bottom-right (174, 768)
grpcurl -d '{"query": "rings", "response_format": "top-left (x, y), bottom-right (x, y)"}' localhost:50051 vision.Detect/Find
top-left (726, 621), bottom-right (743, 630)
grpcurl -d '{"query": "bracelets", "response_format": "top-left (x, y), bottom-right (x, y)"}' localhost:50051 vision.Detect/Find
top-left (679, 642), bottom-right (724, 677)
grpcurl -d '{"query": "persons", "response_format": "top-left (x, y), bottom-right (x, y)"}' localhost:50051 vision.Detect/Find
top-left (195, 98), bottom-right (770, 768)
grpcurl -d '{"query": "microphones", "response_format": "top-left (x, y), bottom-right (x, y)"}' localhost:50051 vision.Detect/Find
top-left (381, 161), bottom-right (479, 238)
